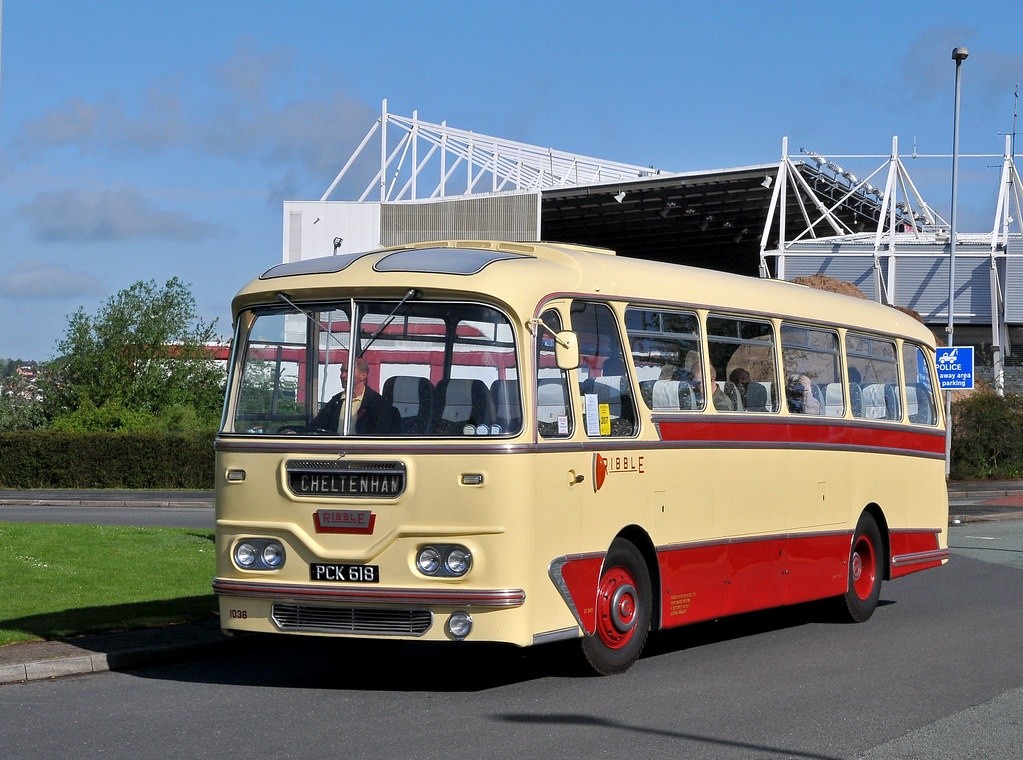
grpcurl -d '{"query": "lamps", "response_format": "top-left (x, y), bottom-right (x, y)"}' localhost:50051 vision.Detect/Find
top-left (761, 172), bottom-right (773, 190)
top-left (614, 188), bottom-right (626, 204)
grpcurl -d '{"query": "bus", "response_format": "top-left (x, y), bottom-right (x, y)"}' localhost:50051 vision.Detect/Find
top-left (210, 234), bottom-right (954, 677)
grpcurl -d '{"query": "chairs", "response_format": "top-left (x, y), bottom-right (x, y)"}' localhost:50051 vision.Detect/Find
top-left (382, 376), bottom-right (570, 434)
top-left (896, 384), bottom-right (934, 425)
top-left (639, 380), bottom-right (696, 410)
top-left (747, 382), bottom-right (778, 411)
top-left (716, 381), bottom-right (741, 410)
top-left (820, 383), bottom-right (864, 416)
top-left (861, 384), bottom-right (898, 420)
top-left (581, 374), bottom-right (631, 422)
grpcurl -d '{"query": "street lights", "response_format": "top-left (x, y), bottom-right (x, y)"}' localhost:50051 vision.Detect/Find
top-left (944, 46), bottom-right (970, 481)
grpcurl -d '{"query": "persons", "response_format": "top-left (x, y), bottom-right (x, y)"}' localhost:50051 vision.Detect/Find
top-left (773, 370), bottom-right (820, 413)
top-left (727, 367), bottom-right (754, 412)
top-left (677, 359), bottom-right (734, 412)
top-left (287, 356), bottom-right (400, 435)
